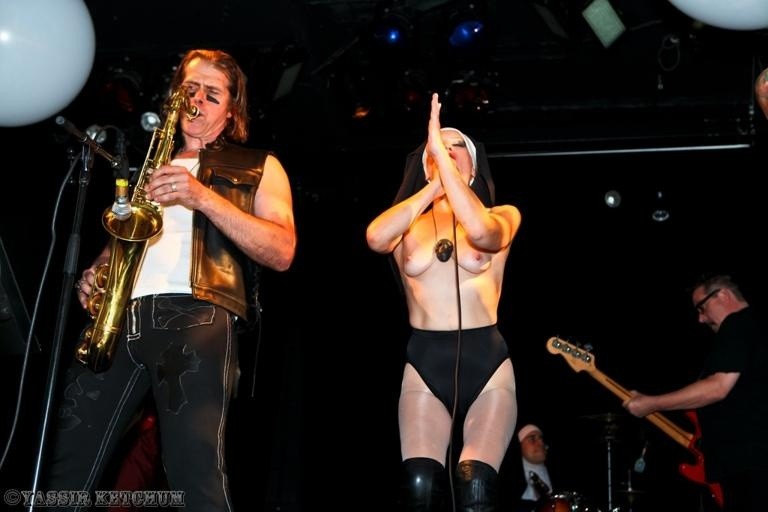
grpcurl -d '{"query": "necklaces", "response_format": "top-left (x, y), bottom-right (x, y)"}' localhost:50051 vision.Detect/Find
top-left (432, 208), bottom-right (457, 263)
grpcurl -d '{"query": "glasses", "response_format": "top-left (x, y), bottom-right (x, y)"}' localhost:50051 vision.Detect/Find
top-left (696, 288), bottom-right (720, 313)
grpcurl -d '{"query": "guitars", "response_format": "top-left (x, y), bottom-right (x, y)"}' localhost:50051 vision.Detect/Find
top-left (546, 336), bottom-right (724, 506)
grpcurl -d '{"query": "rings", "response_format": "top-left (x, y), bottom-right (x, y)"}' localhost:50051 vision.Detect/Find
top-left (170, 182), bottom-right (177, 192)
top-left (75, 279), bottom-right (88, 293)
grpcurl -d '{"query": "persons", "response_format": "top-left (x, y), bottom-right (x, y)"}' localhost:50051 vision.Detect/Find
top-left (620, 277), bottom-right (767, 511)
top-left (364, 92), bottom-right (522, 512)
top-left (492, 424), bottom-right (556, 512)
top-left (36, 48), bottom-right (299, 511)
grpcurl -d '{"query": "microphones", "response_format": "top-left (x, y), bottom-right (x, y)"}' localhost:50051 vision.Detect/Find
top-left (111, 119), bottom-right (132, 221)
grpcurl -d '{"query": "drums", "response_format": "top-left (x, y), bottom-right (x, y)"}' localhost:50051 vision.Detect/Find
top-left (537, 495), bottom-right (578, 511)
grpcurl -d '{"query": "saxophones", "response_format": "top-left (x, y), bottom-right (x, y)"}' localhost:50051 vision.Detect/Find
top-left (75, 86), bottom-right (200, 374)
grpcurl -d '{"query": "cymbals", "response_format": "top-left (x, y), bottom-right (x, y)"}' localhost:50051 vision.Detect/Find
top-left (578, 412), bottom-right (629, 430)
